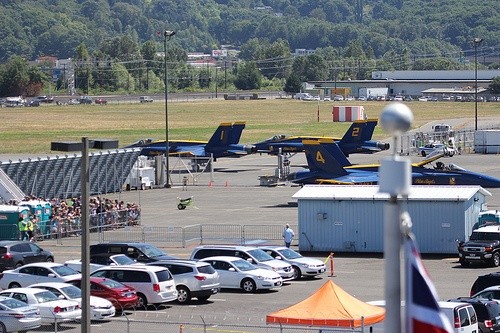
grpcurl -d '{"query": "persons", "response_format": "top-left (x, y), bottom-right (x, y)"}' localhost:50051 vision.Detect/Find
top-left (0, 195), bottom-right (142, 241)
top-left (282, 224), bottom-right (295, 248)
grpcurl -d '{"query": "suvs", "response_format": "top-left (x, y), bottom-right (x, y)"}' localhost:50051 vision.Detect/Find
top-left (0, 241), bottom-right (55, 270)
top-left (64, 254), bottom-right (221, 308)
top-left (190, 246), bottom-right (295, 282)
top-left (139, 96), bottom-right (153, 103)
top-left (89, 244), bottom-right (182, 269)
top-left (362, 207), bottom-right (500, 333)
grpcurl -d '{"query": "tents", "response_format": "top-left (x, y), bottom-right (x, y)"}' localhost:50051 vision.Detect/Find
top-left (266, 279), bottom-right (386, 333)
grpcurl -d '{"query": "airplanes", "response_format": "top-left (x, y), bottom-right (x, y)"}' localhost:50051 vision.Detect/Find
top-left (119, 120), bottom-right (500, 195)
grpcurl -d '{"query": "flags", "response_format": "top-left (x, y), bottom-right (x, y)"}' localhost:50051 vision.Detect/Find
top-left (405, 234), bottom-right (453, 333)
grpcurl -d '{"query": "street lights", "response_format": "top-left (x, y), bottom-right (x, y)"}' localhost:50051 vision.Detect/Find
top-left (473, 38), bottom-right (484, 131)
top-left (164, 30), bottom-right (179, 189)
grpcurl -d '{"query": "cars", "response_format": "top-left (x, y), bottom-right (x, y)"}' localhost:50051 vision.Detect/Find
top-left (0, 263), bottom-right (83, 290)
top-left (419, 142), bottom-right (455, 158)
top-left (1, 296), bottom-right (42, 333)
top-left (199, 256), bottom-right (283, 292)
top-left (290, 91), bottom-right (500, 102)
top-left (14, 283), bottom-right (114, 318)
top-left (259, 246), bottom-right (327, 279)
top-left (63, 277), bottom-right (138, 312)
top-left (0, 288), bottom-right (83, 325)
top-left (0, 96), bottom-right (54, 107)
top-left (68, 96), bottom-right (107, 106)
